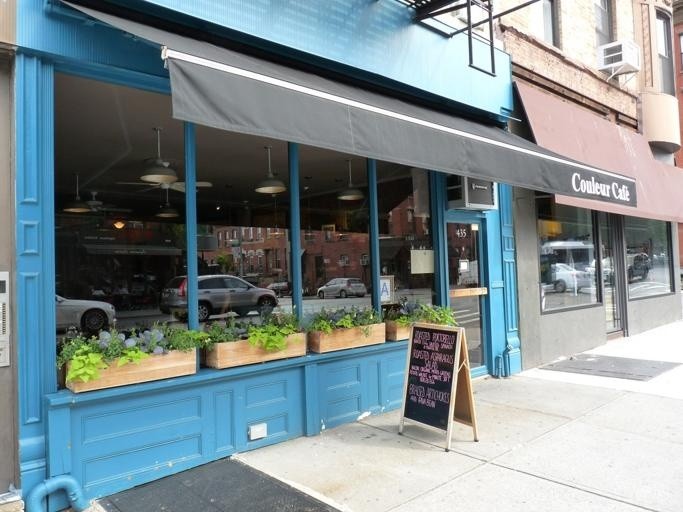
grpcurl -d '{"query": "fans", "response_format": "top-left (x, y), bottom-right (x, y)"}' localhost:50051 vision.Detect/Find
top-left (84, 191), bottom-right (133, 213)
top-left (114, 161), bottom-right (213, 194)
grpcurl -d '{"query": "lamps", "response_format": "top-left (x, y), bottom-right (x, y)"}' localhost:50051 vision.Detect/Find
top-left (254, 145), bottom-right (286, 195)
top-left (154, 190), bottom-right (180, 218)
top-left (62, 172), bottom-right (91, 213)
top-left (140, 126), bottom-right (178, 185)
top-left (337, 159), bottom-right (365, 201)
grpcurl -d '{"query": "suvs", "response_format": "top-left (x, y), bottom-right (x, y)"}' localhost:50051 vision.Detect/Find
top-left (159, 274), bottom-right (279, 322)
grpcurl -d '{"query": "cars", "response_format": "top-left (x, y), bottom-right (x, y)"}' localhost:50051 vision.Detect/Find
top-left (266, 282), bottom-right (292, 298)
top-left (537, 238), bottom-right (651, 293)
top-left (316, 277), bottom-right (367, 299)
top-left (55, 294), bottom-right (116, 339)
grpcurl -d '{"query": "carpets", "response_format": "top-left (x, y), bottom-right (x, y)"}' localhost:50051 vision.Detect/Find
top-left (96, 457), bottom-right (348, 512)
top-left (538, 354), bottom-right (683, 382)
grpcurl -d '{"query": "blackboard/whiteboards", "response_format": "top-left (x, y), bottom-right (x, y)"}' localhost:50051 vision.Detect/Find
top-left (400, 322), bottom-right (462, 435)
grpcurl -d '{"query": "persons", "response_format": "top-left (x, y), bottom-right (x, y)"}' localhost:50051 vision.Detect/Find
top-left (140, 280), bottom-right (156, 307)
top-left (113, 284), bottom-right (129, 294)
